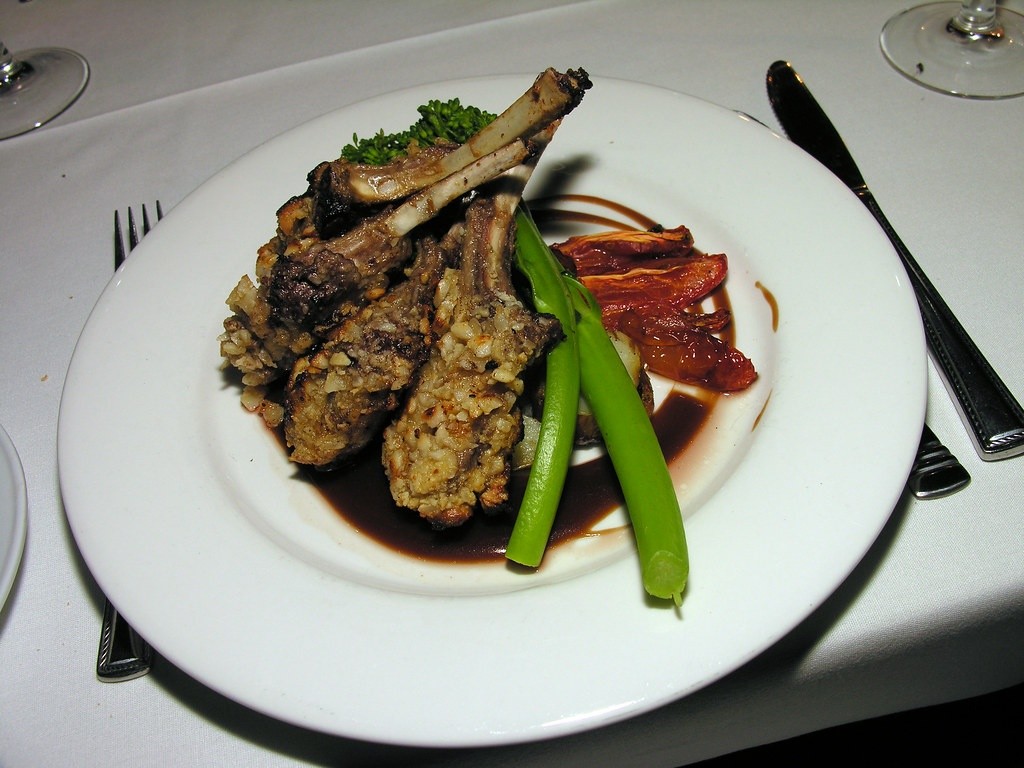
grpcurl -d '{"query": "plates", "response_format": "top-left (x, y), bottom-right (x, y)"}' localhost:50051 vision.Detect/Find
top-left (1, 428), bottom-right (28, 609)
top-left (56, 75), bottom-right (928, 748)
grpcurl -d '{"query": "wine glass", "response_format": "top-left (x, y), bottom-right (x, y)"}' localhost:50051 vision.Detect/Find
top-left (0, 42), bottom-right (90, 141)
top-left (879, 0), bottom-right (1024, 101)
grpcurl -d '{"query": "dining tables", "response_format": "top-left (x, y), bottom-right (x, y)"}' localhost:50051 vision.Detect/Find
top-left (0, 0), bottom-right (1024, 767)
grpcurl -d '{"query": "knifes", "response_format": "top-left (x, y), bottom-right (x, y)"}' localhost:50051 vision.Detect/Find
top-left (766, 60), bottom-right (1024, 462)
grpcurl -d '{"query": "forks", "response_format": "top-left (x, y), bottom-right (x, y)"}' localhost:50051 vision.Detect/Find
top-left (95, 200), bottom-right (164, 683)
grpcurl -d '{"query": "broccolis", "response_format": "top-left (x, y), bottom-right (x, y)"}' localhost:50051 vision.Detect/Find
top-left (337, 95), bottom-right (498, 165)
